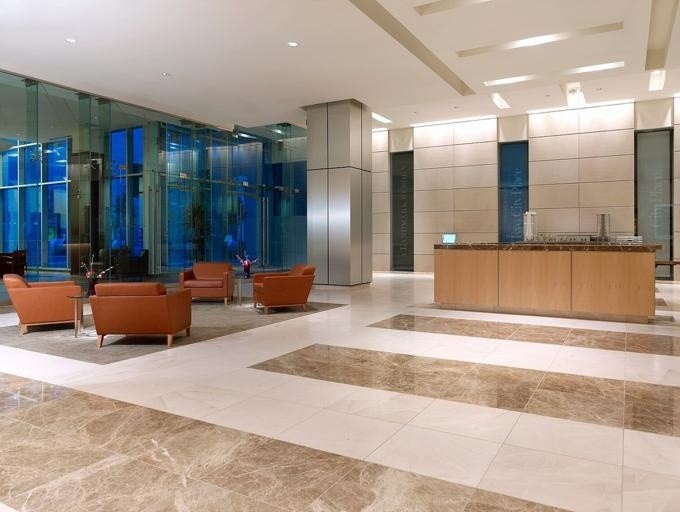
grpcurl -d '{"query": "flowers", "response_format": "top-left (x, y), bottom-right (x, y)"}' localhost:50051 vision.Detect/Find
top-left (80, 254), bottom-right (115, 285)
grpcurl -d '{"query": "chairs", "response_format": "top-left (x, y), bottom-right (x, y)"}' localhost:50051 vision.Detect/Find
top-left (178, 262), bottom-right (315, 315)
top-left (3, 273), bottom-right (192, 348)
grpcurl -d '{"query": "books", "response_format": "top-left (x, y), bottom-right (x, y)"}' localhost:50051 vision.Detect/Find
top-left (616, 235), bottom-right (643, 245)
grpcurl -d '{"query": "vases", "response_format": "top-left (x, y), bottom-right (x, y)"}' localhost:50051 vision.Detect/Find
top-left (86, 279), bottom-right (96, 298)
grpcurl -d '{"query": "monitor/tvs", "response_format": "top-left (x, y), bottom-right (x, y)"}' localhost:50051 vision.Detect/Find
top-left (442, 233), bottom-right (456, 244)
